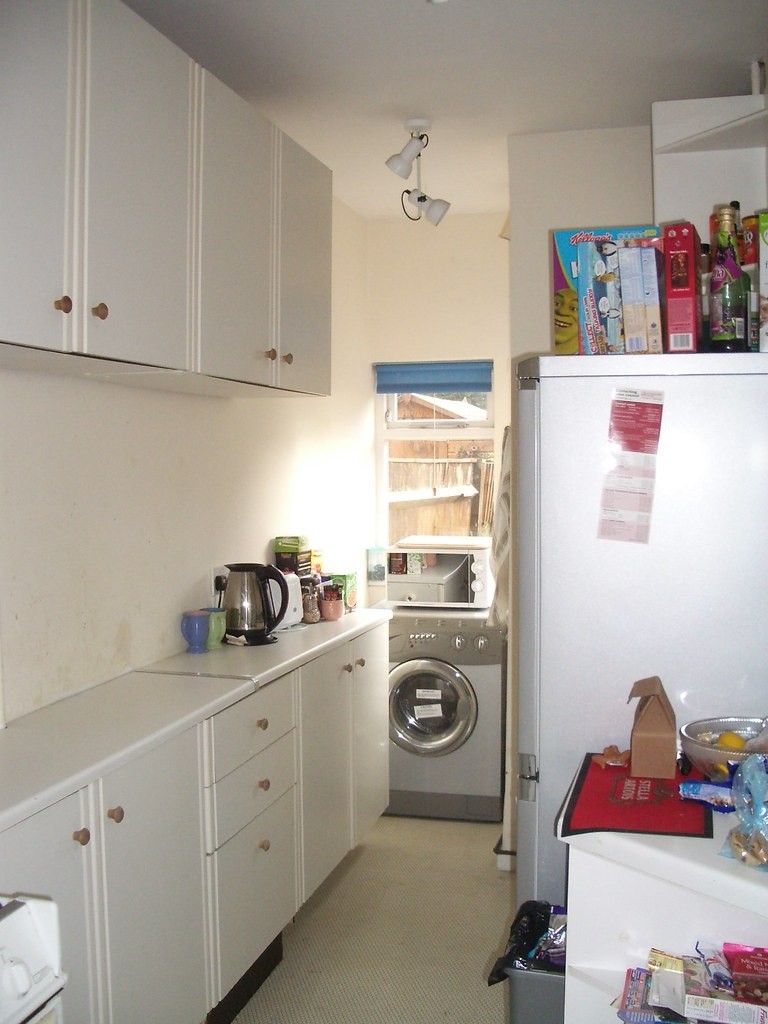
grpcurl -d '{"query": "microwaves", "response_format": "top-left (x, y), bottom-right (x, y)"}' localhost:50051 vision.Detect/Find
top-left (385, 535), bottom-right (496, 609)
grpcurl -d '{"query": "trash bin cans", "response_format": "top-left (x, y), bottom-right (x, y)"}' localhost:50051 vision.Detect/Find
top-left (504, 899), bottom-right (567, 1024)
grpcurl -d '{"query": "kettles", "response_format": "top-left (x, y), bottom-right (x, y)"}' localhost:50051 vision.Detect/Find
top-left (221, 563), bottom-right (289, 646)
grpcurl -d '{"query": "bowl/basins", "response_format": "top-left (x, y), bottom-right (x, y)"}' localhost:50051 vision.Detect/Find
top-left (679, 716), bottom-right (768, 782)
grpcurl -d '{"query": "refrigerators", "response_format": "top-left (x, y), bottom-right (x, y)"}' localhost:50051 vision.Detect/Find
top-left (518, 352), bottom-right (768, 916)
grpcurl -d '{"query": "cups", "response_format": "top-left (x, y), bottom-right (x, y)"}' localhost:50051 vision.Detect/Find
top-left (321, 599), bottom-right (343, 620)
top-left (201, 608), bottom-right (227, 649)
top-left (181, 611), bottom-right (209, 653)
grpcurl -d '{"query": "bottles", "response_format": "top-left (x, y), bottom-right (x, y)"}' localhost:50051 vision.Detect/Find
top-left (700, 200), bottom-right (759, 351)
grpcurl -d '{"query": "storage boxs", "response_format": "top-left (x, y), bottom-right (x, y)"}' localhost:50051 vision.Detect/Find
top-left (759, 213), bottom-right (768, 353)
top-left (553, 226), bottom-right (660, 355)
top-left (275, 535), bottom-right (357, 614)
top-left (617, 246), bottom-right (648, 354)
top-left (641, 246), bottom-right (665, 353)
top-left (664, 222), bottom-right (702, 353)
top-left (577, 238), bottom-right (664, 356)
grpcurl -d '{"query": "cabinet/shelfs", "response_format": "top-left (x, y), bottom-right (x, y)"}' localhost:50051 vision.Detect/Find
top-left (203, 669), bottom-right (300, 1024)
top-left (0, 0), bottom-right (192, 375)
top-left (85, 63), bottom-right (333, 397)
top-left (0, 721), bottom-right (211, 1024)
top-left (296, 619), bottom-right (389, 905)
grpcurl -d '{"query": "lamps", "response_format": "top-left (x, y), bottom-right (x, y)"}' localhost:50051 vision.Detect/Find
top-left (385, 118), bottom-right (450, 226)
